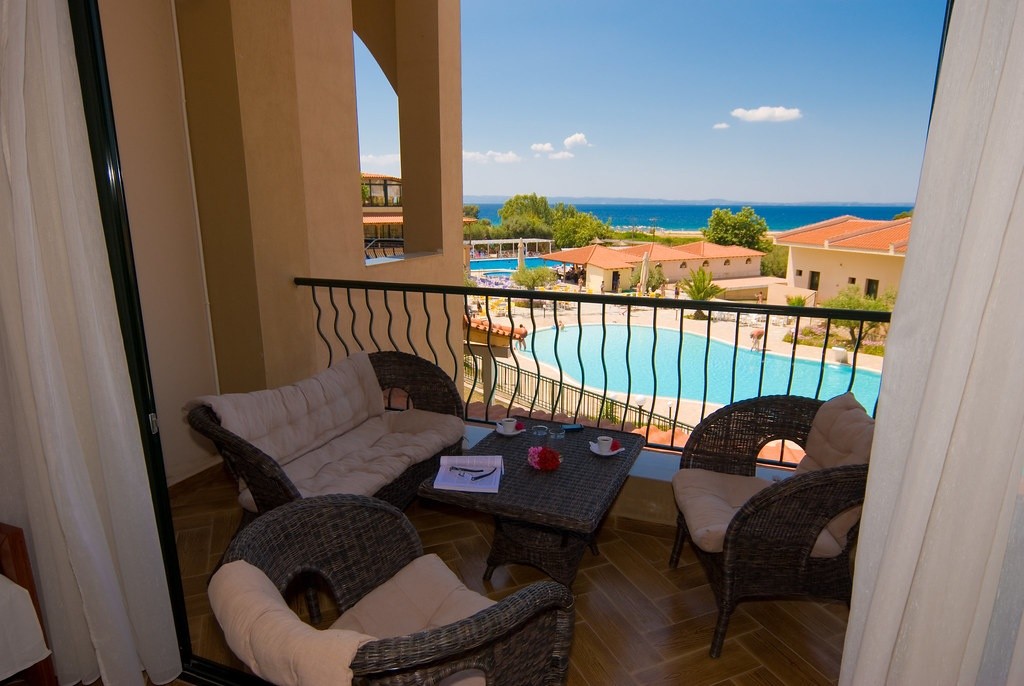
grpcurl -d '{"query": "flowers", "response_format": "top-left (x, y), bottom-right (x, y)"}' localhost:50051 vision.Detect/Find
top-left (515, 422), bottom-right (525, 430)
top-left (527, 446), bottom-right (564, 472)
top-left (611, 440), bottom-right (621, 450)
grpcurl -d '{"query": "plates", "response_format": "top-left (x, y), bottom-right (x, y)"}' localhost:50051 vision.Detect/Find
top-left (496, 428), bottom-right (521, 436)
top-left (590, 444), bottom-right (625, 455)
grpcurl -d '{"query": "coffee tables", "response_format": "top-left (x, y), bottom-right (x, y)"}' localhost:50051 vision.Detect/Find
top-left (419, 415), bottom-right (646, 595)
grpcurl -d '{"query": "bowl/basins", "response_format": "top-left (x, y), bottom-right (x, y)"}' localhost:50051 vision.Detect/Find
top-left (532, 426), bottom-right (565, 463)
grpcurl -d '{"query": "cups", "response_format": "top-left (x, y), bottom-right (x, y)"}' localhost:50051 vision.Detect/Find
top-left (598, 436), bottom-right (612, 452)
top-left (502, 418), bottom-right (516, 432)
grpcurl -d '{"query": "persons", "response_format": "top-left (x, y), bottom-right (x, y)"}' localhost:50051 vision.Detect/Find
top-left (601, 281), bottom-right (605, 294)
top-left (661, 285), bottom-right (666, 296)
top-left (675, 281), bottom-right (679, 299)
top-left (559, 320), bottom-right (564, 330)
top-left (749, 330), bottom-right (764, 351)
top-left (519, 324), bottom-right (526, 351)
top-left (477, 301), bottom-right (482, 311)
top-left (497, 251), bottom-right (516, 258)
top-left (556, 267), bottom-right (586, 285)
top-left (615, 274), bottom-right (621, 293)
top-left (757, 291), bottom-right (763, 304)
top-left (471, 249), bottom-right (486, 258)
top-left (528, 251), bottom-right (549, 257)
top-left (578, 275), bottom-right (583, 293)
top-left (637, 281), bottom-right (641, 296)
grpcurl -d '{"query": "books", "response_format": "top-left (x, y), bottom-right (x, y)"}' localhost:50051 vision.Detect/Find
top-left (434, 456), bottom-right (504, 493)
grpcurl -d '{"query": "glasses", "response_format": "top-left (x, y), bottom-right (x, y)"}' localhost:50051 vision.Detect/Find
top-left (449, 465), bottom-right (497, 482)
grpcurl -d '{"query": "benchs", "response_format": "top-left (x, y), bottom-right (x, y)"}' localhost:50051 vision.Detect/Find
top-left (180, 350), bottom-right (465, 553)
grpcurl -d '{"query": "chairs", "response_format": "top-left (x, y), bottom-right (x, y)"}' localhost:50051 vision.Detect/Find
top-left (671, 391), bottom-right (874, 658)
top-left (205, 493), bottom-right (575, 686)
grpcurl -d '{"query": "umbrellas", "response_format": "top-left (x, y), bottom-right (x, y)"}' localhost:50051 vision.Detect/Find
top-left (641, 252), bottom-right (649, 296)
top-left (518, 238), bottom-right (524, 268)
